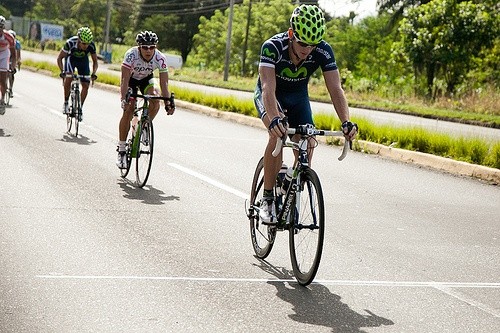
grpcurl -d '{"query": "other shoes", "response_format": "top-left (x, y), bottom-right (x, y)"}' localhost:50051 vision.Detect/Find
top-left (0, 100), bottom-right (6, 115)
top-left (9, 89), bottom-right (14, 98)
top-left (63, 102), bottom-right (70, 113)
top-left (116, 145), bottom-right (126, 162)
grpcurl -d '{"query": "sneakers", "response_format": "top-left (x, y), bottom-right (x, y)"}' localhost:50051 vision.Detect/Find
top-left (260, 197), bottom-right (277, 224)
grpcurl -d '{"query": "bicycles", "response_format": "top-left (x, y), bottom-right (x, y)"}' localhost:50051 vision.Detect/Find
top-left (5, 62), bottom-right (21, 105)
top-left (61, 71), bottom-right (95, 139)
top-left (243, 116), bottom-right (354, 287)
top-left (116, 87), bottom-right (175, 189)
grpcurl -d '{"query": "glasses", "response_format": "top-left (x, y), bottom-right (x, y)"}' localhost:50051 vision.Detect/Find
top-left (0, 26), bottom-right (2, 28)
top-left (140, 45), bottom-right (156, 50)
top-left (292, 33), bottom-right (317, 48)
top-left (82, 43), bottom-right (86, 45)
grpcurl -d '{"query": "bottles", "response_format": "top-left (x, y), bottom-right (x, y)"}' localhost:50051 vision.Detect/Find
top-left (280, 166), bottom-right (294, 195)
top-left (276, 161), bottom-right (287, 196)
top-left (133, 121), bottom-right (140, 138)
top-left (132, 113), bottom-right (138, 135)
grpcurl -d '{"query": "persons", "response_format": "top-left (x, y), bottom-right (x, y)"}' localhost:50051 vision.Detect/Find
top-left (118, 31), bottom-right (174, 168)
top-left (57, 26), bottom-right (98, 118)
top-left (28, 21), bottom-right (41, 47)
top-left (253, 4), bottom-right (358, 234)
top-left (7, 29), bottom-right (20, 96)
top-left (0, 15), bottom-right (16, 105)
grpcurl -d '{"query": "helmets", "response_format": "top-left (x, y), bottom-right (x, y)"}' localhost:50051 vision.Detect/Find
top-left (136, 30), bottom-right (159, 46)
top-left (77, 27), bottom-right (93, 44)
top-left (8, 30), bottom-right (16, 36)
top-left (290, 4), bottom-right (326, 46)
top-left (0, 16), bottom-right (5, 25)
